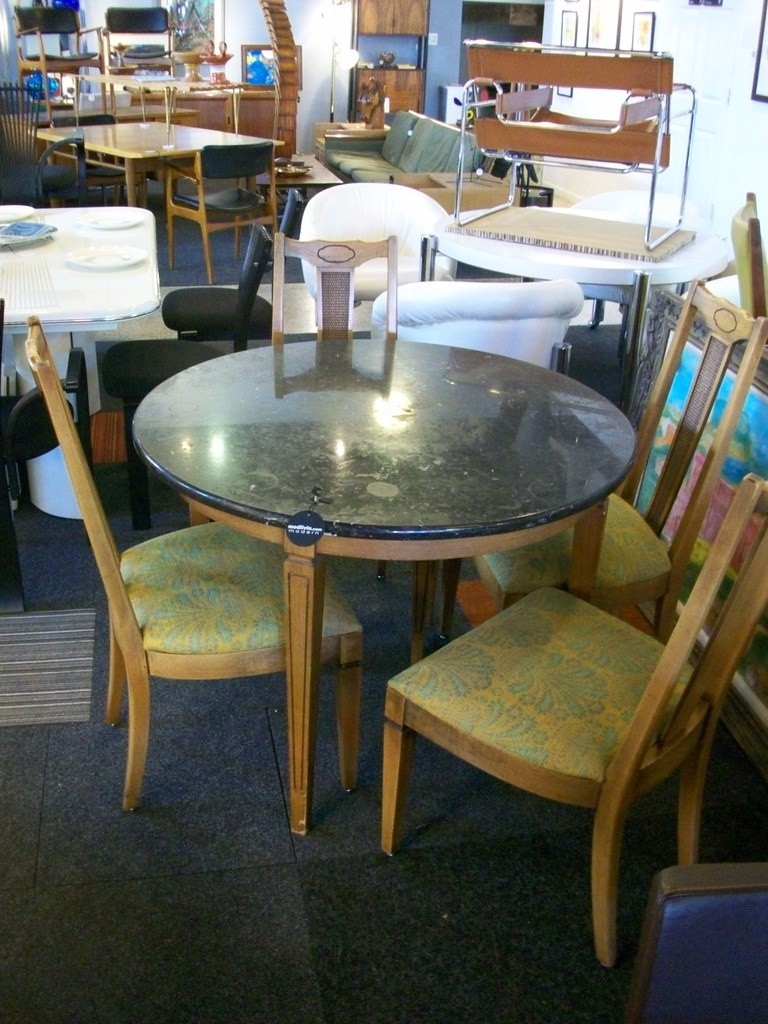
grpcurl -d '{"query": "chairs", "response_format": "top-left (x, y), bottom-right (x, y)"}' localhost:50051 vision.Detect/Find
top-left (103, 6), bottom-right (176, 113)
top-left (0, 142), bottom-right (768, 969)
top-left (13, 6), bottom-right (108, 124)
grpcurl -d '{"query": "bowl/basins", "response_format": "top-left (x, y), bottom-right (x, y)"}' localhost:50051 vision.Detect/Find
top-left (200, 54), bottom-right (234, 65)
top-left (173, 52), bottom-right (206, 64)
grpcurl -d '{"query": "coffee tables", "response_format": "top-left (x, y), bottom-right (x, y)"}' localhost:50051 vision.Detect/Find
top-left (255, 154), bottom-right (343, 220)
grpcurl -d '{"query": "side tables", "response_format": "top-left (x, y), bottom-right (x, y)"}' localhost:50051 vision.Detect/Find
top-left (388, 170), bottom-right (521, 216)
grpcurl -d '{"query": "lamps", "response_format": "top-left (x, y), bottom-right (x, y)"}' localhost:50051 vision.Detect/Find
top-left (327, 42), bottom-right (358, 122)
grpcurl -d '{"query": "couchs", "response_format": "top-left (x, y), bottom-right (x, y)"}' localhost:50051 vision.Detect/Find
top-left (319, 108), bottom-right (482, 188)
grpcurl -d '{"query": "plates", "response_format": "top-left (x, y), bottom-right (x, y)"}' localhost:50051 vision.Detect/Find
top-left (66, 244), bottom-right (148, 269)
top-left (0, 224), bottom-right (58, 245)
top-left (91, 211), bottom-right (144, 229)
top-left (0, 203), bottom-right (35, 223)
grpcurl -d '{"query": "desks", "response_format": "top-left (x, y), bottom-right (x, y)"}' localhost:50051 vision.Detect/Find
top-left (130, 341), bottom-right (637, 829)
top-left (422, 203), bottom-right (730, 366)
top-left (28, 122), bottom-right (286, 254)
top-left (1, 104), bottom-right (201, 180)
top-left (0, 203), bottom-right (159, 397)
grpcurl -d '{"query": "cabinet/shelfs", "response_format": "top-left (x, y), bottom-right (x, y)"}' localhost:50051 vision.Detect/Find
top-left (459, 2), bottom-right (545, 99)
top-left (455, 40), bottom-right (697, 249)
top-left (336, 1), bottom-right (430, 135)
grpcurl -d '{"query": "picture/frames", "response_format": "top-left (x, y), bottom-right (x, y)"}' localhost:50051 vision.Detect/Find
top-left (240, 45), bottom-right (304, 91)
top-left (630, 10), bottom-right (655, 53)
top-left (555, 10), bottom-right (578, 96)
top-left (751, 1), bottom-right (768, 105)
